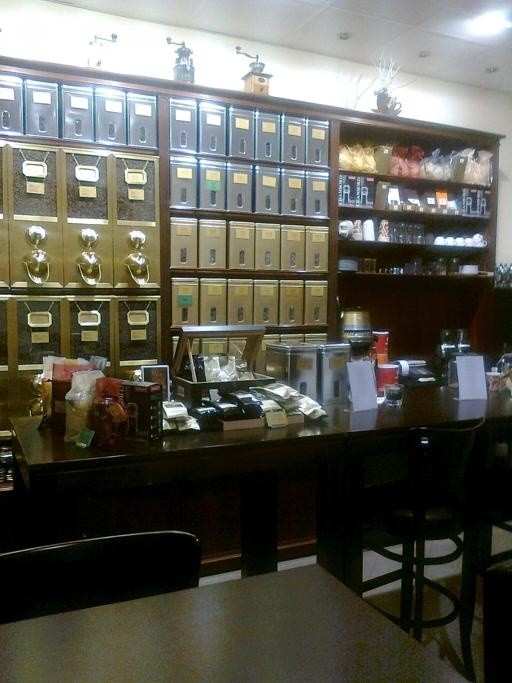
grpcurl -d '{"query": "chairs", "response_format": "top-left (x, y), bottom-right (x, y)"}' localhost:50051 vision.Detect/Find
top-left (0, 530), bottom-right (201, 624)
top-left (345, 417), bottom-right (486, 682)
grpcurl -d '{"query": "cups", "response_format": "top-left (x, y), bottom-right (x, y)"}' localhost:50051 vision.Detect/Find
top-left (351, 219), bottom-right (487, 248)
top-left (376, 363), bottom-right (400, 398)
top-left (383, 383), bottom-right (405, 408)
top-left (371, 330), bottom-right (390, 364)
top-left (404, 254), bottom-right (447, 276)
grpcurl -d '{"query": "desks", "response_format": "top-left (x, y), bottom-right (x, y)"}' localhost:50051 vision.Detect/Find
top-left (0, 563), bottom-right (468, 682)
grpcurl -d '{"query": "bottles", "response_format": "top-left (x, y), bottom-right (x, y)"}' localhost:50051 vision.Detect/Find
top-left (493, 262), bottom-right (512, 288)
top-left (87, 378), bottom-right (130, 452)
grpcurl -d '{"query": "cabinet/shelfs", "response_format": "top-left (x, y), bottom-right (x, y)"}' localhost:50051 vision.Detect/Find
top-left (0, 55), bottom-right (506, 377)
